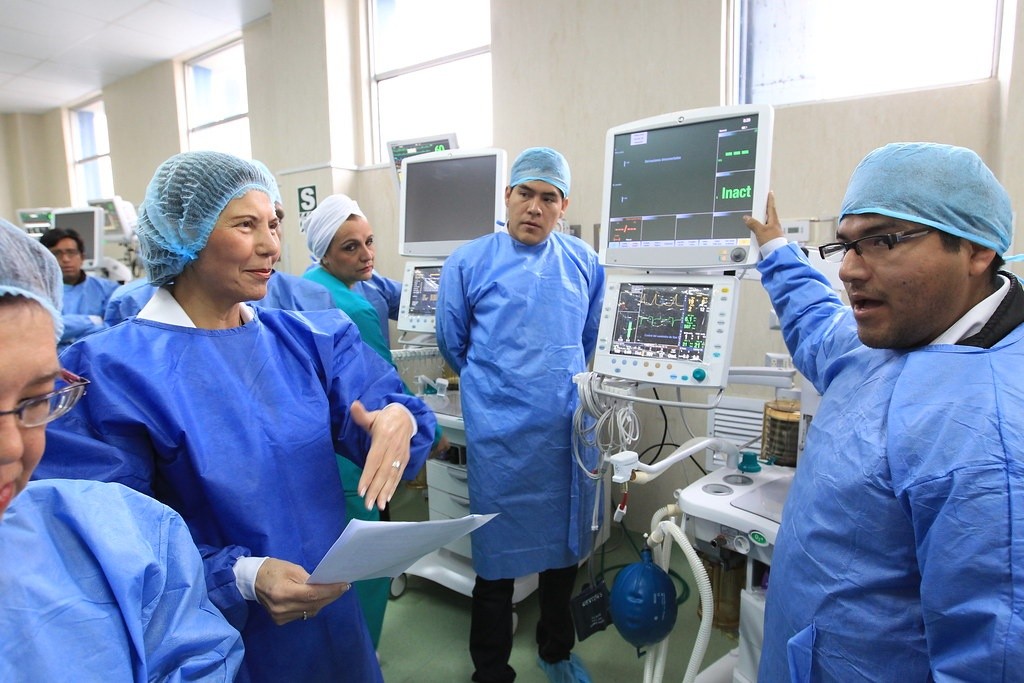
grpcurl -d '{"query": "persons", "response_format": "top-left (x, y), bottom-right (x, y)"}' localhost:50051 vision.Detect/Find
top-left (304, 260), bottom-right (403, 348)
top-left (30, 153), bottom-right (438, 683)
top-left (434, 146), bottom-right (606, 683)
top-left (105, 161), bottom-right (335, 329)
top-left (303, 193), bottom-right (450, 667)
top-left (0, 217), bottom-right (245, 683)
top-left (745, 142), bottom-right (1024, 683)
top-left (40, 227), bottom-right (119, 354)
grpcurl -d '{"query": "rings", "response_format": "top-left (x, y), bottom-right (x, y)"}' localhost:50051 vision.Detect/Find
top-left (304, 610), bottom-right (307, 620)
top-left (392, 461), bottom-right (401, 470)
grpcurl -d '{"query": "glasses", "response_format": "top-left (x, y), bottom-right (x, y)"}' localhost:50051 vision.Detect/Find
top-left (818, 223), bottom-right (935, 264)
top-left (0, 367), bottom-right (91, 428)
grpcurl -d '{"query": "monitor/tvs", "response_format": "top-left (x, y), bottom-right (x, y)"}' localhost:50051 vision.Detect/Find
top-left (599, 103), bottom-right (775, 273)
top-left (18, 207), bottom-right (53, 241)
top-left (88, 196), bottom-right (138, 242)
top-left (52, 207), bottom-right (104, 270)
top-left (396, 258), bottom-right (444, 334)
top-left (593, 272), bottom-right (740, 390)
top-left (398, 147), bottom-right (507, 256)
